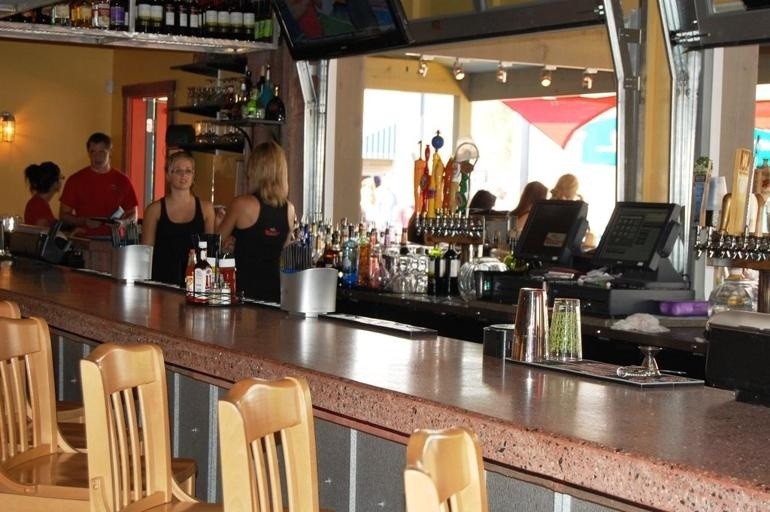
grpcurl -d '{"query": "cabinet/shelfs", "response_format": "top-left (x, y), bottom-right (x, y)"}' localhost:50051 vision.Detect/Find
top-left (164, 60), bottom-right (279, 156)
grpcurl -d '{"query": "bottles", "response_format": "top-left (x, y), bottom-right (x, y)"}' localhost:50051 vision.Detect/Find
top-left (185, 238), bottom-right (237, 307)
top-left (280, 208), bottom-right (509, 302)
top-left (187, 63), bottom-right (287, 121)
top-left (13, 1), bottom-right (275, 43)
top-left (192, 119), bottom-right (243, 145)
top-left (184, 307), bottom-right (240, 342)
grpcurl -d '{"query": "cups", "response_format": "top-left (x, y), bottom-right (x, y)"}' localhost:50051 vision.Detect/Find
top-left (511, 287), bottom-right (584, 364)
top-left (509, 362), bottom-right (584, 424)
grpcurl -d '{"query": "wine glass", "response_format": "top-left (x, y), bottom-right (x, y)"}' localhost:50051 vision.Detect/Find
top-left (639, 346), bottom-right (663, 378)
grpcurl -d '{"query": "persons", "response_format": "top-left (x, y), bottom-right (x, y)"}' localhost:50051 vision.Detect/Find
top-left (59, 132), bottom-right (138, 236)
top-left (551, 174), bottom-right (578, 200)
top-left (469, 190), bottom-right (497, 211)
top-left (694, 157), bottom-right (714, 226)
top-left (141, 153), bottom-right (215, 284)
top-left (24, 161), bottom-right (64, 229)
top-left (508, 181), bottom-right (551, 240)
top-left (214, 141), bottom-right (294, 304)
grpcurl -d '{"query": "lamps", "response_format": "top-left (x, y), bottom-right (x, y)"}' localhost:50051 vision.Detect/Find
top-left (418, 54), bottom-right (597, 92)
top-left (1, 110), bottom-right (18, 146)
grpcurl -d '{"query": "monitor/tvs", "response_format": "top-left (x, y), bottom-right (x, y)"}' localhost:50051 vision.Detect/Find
top-left (271, 0), bottom-right (414, 61)
top-left (513, 200), bottom-right (588, 264)
top-left (591, 201), bottom-right (681, 272)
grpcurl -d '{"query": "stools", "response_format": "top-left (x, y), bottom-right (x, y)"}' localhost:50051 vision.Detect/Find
top-left (219, 375), bottom-right (320, 511)
top-left (2, 298), bottom-right (196, 510)
top-left (402, 426), bottom-right (488, 512)
top-left (80, 342), bottom-right (222, 511)
